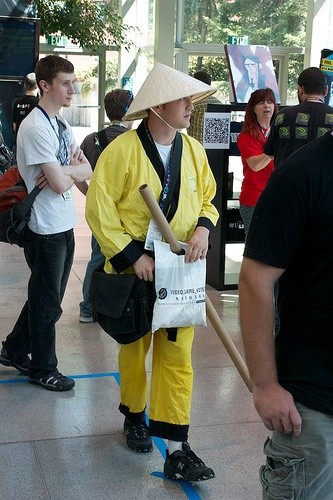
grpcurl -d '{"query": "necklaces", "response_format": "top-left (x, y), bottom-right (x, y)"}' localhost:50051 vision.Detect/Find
top-left (304, 96), bottom-right (323, 103)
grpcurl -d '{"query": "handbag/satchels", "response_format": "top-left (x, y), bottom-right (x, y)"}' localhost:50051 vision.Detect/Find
top-left (150, 240), bottom-right (208, 333)
top-left (0, 164), bottom-right (36, 248)
top-left (89, 260), bottom-right (156, 344)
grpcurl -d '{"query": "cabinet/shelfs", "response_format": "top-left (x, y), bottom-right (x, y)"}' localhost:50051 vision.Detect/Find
top-left (205, 121), bottom-right (246, 291)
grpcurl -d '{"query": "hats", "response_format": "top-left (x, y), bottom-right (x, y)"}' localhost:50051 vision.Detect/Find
top-left (122, 62), bottom-right (216, 121)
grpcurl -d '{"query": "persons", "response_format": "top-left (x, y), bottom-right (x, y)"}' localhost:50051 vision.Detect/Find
top-left (237, 130), bottom-right (333, 500)
top-left (185, 71), bottom-right (221, 148)
top-left (13, 72), bottom-right (43, 140)
top-left (0, 55), bottom-right (93, 392)
top-left (78, 90), bottom-right (136, 324)
top-left (264, 67), bottom-right (333, 171)
top-left (86, 61), bottom-right (222, 482)
top-left (238, 88), bottom-right (279, 245)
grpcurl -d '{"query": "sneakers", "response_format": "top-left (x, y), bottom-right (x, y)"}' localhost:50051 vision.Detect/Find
top-left (123, 416), bottom-right (153, 453)
top-left (28, 367), bottom-right (75, 391)
top-left (163, 441), bottom-right (215, 482)
top-left (0, 340), bottom-right (31, 376)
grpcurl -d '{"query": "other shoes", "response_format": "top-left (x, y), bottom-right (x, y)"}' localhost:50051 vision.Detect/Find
top-left (79, 315), bottom-right (93, 322)
